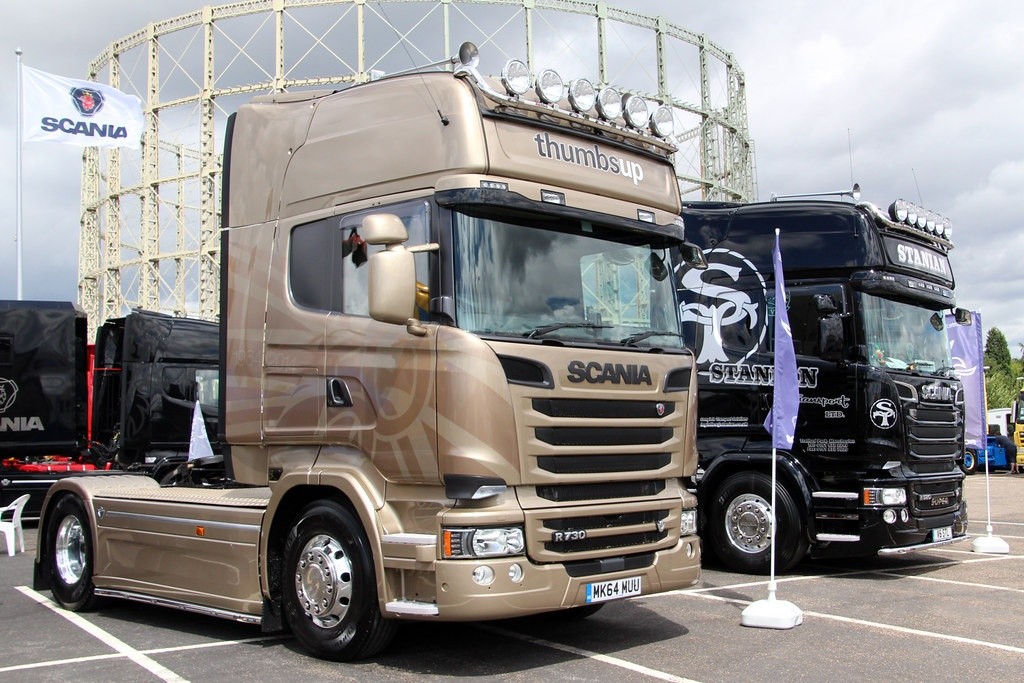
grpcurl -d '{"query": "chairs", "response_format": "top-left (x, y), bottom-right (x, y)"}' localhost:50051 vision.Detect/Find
top-left (0, 494), bottom-right (30, 557)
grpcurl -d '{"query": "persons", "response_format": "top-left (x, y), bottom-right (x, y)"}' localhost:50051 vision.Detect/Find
top-left (994, 432), bottom-right (1017, 474)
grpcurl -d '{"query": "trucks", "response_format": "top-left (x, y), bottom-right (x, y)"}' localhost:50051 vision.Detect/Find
top-left (670, 182), bottom-right (969, 577)
top-left (959, 376), bottom-right (1024, 476)
top-left (31, 40), bottom-right (709, 663)
top-left (0, 300), bottom-right (232, 521)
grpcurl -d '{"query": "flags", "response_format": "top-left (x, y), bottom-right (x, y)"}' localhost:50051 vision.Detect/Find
top-left (944, 309), bottom-right (988, 451)
top-left (763, 228), bottom-right (800, 450)
top-left (20, 64), bottom-right (147, 150)
